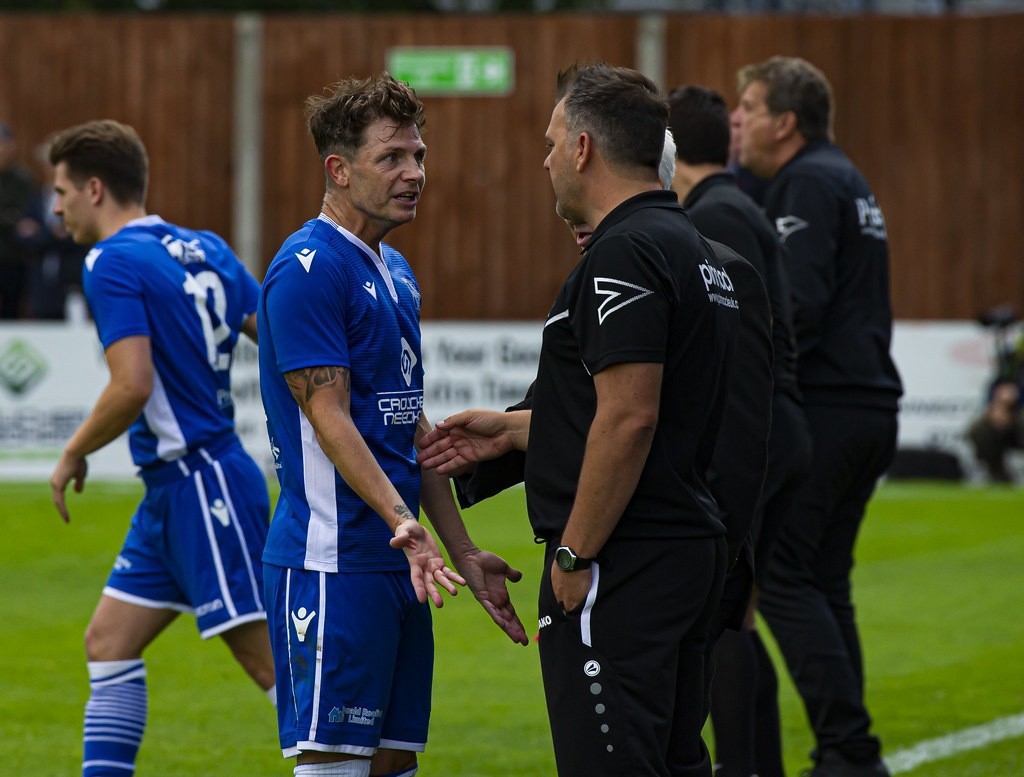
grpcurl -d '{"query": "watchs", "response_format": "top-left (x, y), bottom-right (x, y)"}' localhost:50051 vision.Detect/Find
top-left (552, 543), bottom-right (595, 573)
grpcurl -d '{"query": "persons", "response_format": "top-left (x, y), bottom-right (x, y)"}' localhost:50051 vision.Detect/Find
top-left (0, 126), bottom-right (44, 320)
top-left (46, 119), bottom-right (276, 777)
top-left (254, 71), bottom-right (529, 777)
top-left (725, 57), bottom-right (904, 777)
top-left (656, 80), bottom-right (814, 775)
top-left (955, 371), bottom-right (1023, 486)
top-left (453, 117), bottom-right (773, 738)
top-left (418, 60), bottom-right (740, 776)
top-left (18, 138), bottom-right (96, 322)
